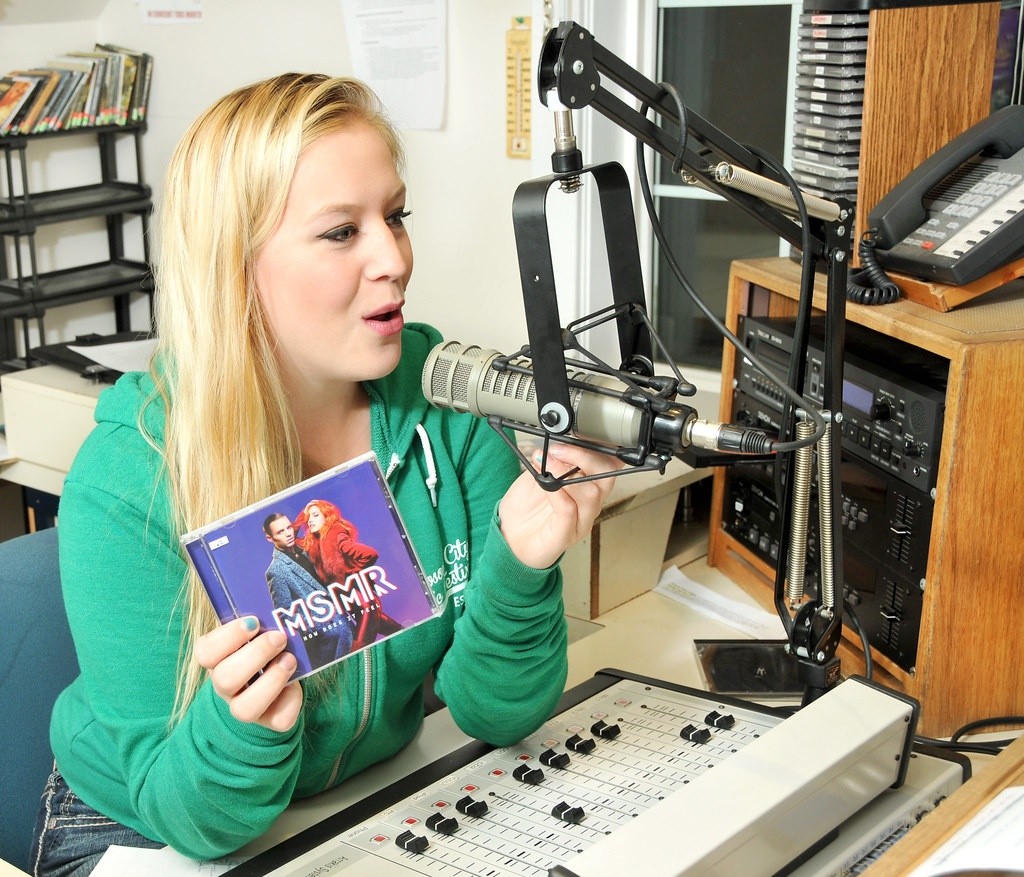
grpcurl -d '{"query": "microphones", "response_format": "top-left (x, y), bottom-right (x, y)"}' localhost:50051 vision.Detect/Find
top-left (420, 341), bottom-right (696, 469)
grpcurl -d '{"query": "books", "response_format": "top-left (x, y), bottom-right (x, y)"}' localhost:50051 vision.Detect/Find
top-left (0, 42), bottom-right (154, 140)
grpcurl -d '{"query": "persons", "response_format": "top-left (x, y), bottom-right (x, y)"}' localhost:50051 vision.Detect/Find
top-left (21, 70), bottom-right (633, 876)
top-left (261, 511), bottom-right (354, 673)
top-left (289, 498), bottom-right (406, 654)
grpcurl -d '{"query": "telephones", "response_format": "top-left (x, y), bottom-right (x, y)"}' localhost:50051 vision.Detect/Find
top-left (865, 98), bottom-right (1024, 288)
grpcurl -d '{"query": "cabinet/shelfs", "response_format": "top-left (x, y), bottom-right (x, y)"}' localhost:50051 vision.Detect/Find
top-left (0, 42), bottom-right (156, 347)
